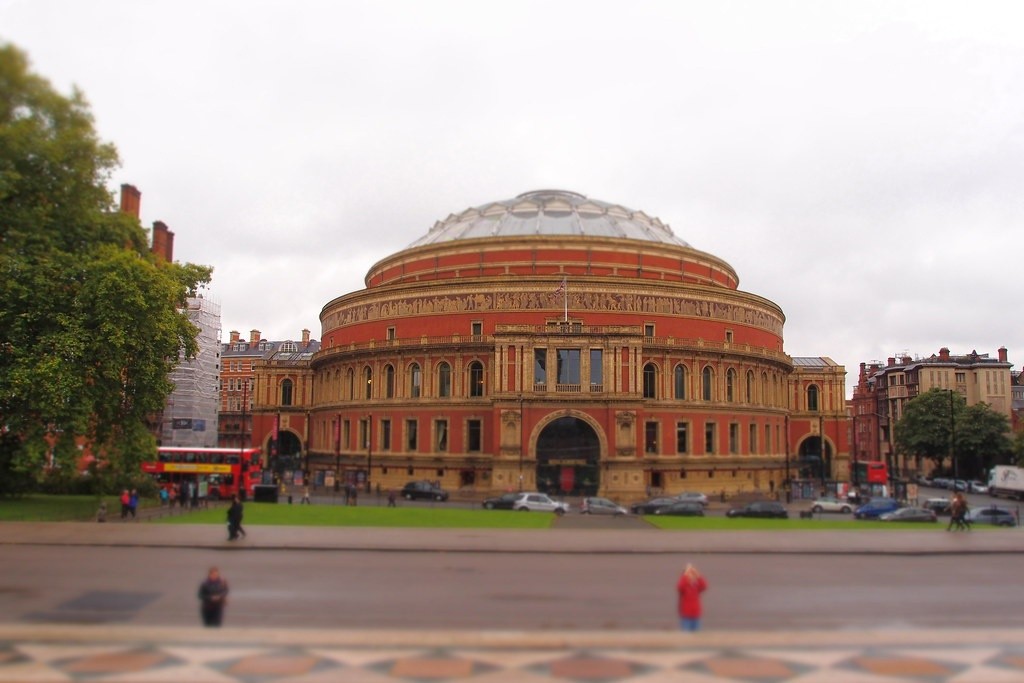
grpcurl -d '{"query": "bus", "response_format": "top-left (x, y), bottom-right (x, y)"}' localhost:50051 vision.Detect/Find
top-left (79, 439), bottom-right (266, 504)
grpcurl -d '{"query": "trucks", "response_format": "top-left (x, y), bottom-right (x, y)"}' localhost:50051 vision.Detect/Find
top-left (988, 464), bottom-right (1024, 503)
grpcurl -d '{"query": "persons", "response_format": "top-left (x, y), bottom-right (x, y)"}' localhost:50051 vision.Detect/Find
top-left (225, 494), bottom-right (246, 541)
top-left (344, 484), bottom-right (358, 506)
top-left (197, 566), bottom-right (228, 628)
top-left (301, 485), bottom-right (310, 504)
top-left (158, 477), bottom-right (209, 511)
top-left (943, 490), bottom-right (971, 532)
top-left (676, 568), bottom-right (708, 632)
top-left (387, 488), bottom-right (396, 507)
top-left (120, 489), bottom-right (138, 520)
top-left (95, 502), bottom-right (107, 523)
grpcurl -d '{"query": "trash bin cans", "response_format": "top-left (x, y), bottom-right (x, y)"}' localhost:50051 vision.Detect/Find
top-left (253, 485), bottom-right (280, 503)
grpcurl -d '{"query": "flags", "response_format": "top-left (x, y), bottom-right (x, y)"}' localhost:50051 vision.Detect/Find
top-left (554, 280), bottom-right (565, 297)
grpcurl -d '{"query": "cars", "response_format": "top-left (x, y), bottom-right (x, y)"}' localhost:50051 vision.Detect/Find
top-left (513, 490), bottom-right (570, 516)
top-left (854, 497), bottom-right (901, 521)
top-left (878, 505), bottom-right (938, 524)
top-left (400, 479), bottom-right (449, 503)
top-left (888, 470), bottom-right (990, 495)
top-left (808, 496), bottom-right (858, 515)
top-left (630, 490), bottom-right (709, 517)
top-left (581, 496), bottom-right (628, 517)
top-left (726, 499), bottom-right (789, 520)
top-left (959, 504), bottom-right (1020, 528)
top-left (923, 498), bottom-right (953, 517)
top-left (482, 492), bottom-right (517, 510)
